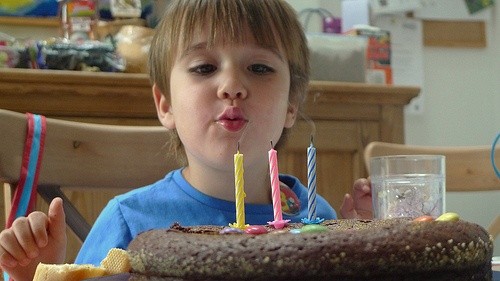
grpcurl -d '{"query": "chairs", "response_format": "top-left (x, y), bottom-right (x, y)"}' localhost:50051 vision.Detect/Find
top-left (364, 142), bottom-right (499, 255)
top-left (0, 109), bottom-right (188, 281)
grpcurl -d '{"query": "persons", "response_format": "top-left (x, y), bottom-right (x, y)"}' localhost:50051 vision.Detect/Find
top-left (0, 0), bottom-right (375, 281)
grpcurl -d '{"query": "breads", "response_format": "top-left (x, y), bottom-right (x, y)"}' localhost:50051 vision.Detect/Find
top-left (32, 261), bottom-right (106, 281)
top-left (101, 248), bottom-right (130, 275)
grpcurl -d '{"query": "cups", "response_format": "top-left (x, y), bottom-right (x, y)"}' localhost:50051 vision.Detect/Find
top-left (364, 154), bottom-right (447, 223)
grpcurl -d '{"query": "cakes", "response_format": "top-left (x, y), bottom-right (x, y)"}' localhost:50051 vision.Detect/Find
top-left (126, 212), bottom-right (493, 281)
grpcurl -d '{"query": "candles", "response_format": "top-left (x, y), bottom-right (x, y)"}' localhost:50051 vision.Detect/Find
top-left (307, 136), bottom-right (316, 220)
top-left (233, 141), bottom-right (248, 229)
top-left (267, 140), bottom-right (282, 223)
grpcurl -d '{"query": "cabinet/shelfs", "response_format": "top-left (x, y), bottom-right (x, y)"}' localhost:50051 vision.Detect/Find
top-left (1, 67), bottom-right (421, 223)
